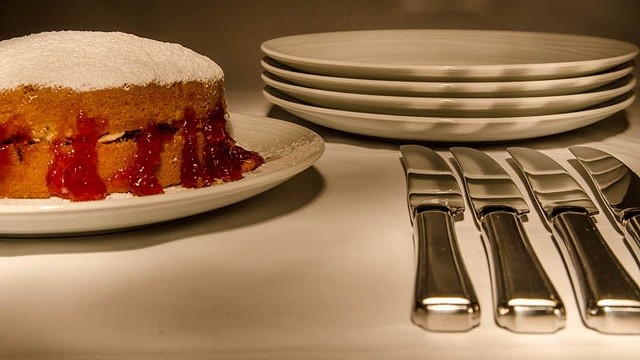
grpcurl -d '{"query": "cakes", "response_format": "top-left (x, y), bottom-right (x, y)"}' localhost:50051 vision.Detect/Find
top-left (1, 30), bottom-right (265, 202)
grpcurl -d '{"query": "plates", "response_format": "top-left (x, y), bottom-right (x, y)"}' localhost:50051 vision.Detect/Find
top-left (1, 111), bottom-right (326, 241)
top-left (260, 27), bottom-right (640, 145)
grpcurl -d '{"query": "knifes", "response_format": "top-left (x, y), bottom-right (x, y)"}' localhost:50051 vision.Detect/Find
top-left (399, 144), bottom-right (481, 332)
top-left (504, 146), bottom-right (640, 333)
top-left (449, 145), bottom-right (567, 334)
top-left (569, 145), bottom-right (640, 271)
